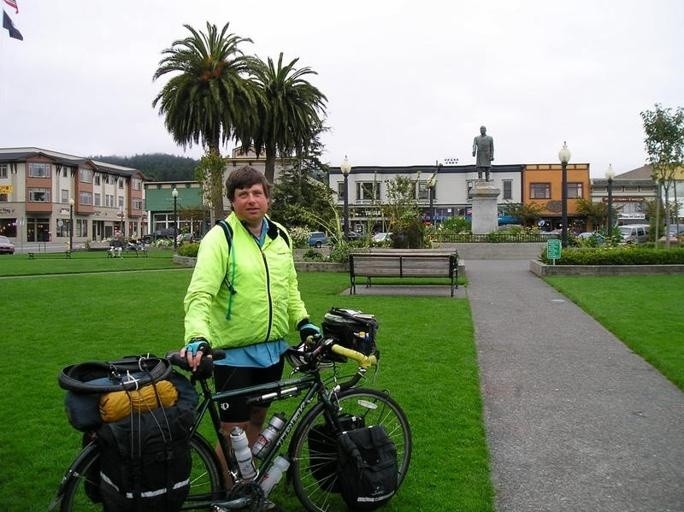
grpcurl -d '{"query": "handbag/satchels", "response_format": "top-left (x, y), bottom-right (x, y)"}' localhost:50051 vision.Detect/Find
top-left (322, 307), bottom-right (379, 356)
top-left (96, 437), bottom-right (193, 512)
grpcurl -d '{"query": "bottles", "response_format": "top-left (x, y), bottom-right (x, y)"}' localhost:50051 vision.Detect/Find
top-left (250, 409), bottom-right (286, 459)
top-left (229, 425), bottom-right (257, 479)
top-left (258, 451), bottom-right (290, 496)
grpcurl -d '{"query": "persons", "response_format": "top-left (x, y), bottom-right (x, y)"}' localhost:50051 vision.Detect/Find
top-left (472, 126), bottom-right (496, 181)
top-left (179, 164), bottom-right (320, 510)
top-left (108, 226), bottom-right (195, 258)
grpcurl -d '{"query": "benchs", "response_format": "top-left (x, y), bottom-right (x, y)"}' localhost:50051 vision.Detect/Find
top-left (109, 241), bottom-right (148, 259)
top-left (346, 246), bottom-right (461, 297)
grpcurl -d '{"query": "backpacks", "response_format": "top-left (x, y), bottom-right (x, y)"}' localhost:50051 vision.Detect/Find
top-left (336, 424), bottom-right (399, 512)
top-left (307, 412), bottom-right (366, 493)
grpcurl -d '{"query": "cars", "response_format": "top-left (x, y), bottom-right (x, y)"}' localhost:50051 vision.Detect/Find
top-left (0, 236), bottom-right (15, 254)
top-left (308, 230), bottom-right (326, 248)
top-left (143, 228), bottom-right (173, 244)
top-left (350, 231), bottom-right (393, 243)
top-left (665, 222), bottom-right (684, 236)
top-left (579, 231), bottom-right (605, 244)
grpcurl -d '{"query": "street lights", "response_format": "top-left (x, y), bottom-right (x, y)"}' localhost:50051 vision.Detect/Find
top-left (559, 141), bottom-right (570, 247)
top-left (340, 156), bottom-right (351, 240)
top-left (67, 198), bottom-right (75, 250)
top-left (604, 162), bottom-right (615, 236)
top-left (172, 187), bottom-right (179, 248)
top-left (428, 176), bottom-right (435, 223)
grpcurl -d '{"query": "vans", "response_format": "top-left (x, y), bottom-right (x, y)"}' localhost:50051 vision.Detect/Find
top-left (618, 224), bottom-right (651, 244)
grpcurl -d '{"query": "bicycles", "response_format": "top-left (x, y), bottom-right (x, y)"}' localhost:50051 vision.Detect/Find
top-left (54, 333), bottom-right (412, 512)
